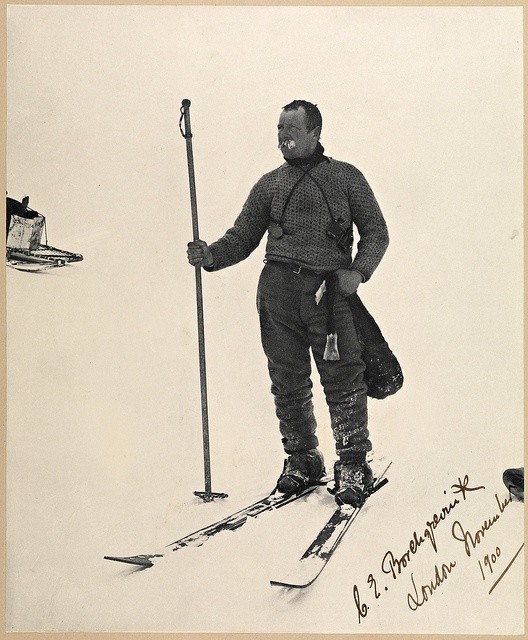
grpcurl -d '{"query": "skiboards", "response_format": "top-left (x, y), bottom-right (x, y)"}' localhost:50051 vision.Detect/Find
top-left (6, 244), bottom-right (83, 272)
top-left (104, 449), bottom-right (391, 589)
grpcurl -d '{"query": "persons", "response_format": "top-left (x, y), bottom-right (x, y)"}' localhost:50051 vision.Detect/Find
top-left (186, 100), bottom-right (390, 509)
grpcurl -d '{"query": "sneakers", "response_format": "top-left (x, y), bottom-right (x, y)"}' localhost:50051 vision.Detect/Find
top-left (277, 449), bottom-right (326, 493)
top-left (326, 460), bottom-right (373, 507)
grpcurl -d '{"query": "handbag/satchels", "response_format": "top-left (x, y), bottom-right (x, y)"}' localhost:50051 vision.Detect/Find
top-left (314, 274), bottom-right (404, 399)
